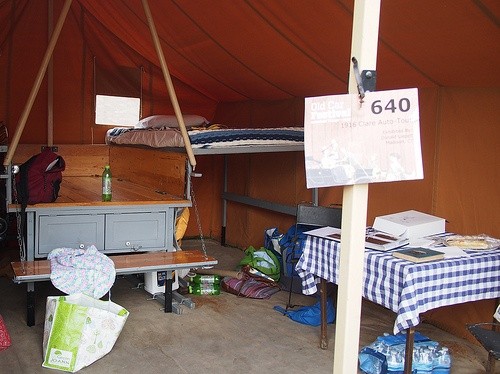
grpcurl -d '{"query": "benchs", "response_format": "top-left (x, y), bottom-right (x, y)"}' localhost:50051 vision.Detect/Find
top-left (9, 249), bottom-right (225, 325)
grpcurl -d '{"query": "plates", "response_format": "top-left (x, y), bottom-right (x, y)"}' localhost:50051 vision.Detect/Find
top-left (443, 234), bottom-right (500, 251)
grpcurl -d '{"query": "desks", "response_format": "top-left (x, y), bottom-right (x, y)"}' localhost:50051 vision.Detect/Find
top-left (7, 174), bottom-right (192, 313)
top-left (295, 235), bottom-right (500, 374)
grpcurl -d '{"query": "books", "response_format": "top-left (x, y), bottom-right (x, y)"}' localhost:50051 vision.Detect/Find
top-left (392, 247), bottom-right (445, 264)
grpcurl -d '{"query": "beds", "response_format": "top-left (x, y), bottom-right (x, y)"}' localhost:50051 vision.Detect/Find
top-left (105, 128), bottom-right (319, 248)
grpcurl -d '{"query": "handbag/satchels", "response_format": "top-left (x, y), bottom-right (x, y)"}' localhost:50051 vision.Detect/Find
top-left (42, 288), bottom-right (129, 373)
top-left (273, 299), bottom-right (335, 327)
top-left (358, 331), bottom-right (450, 374)
top-left (224, 227), bottom-right (307, 299)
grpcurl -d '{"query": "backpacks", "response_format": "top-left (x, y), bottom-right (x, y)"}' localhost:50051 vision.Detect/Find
top-left (16, 148), bottom-right (67, 205)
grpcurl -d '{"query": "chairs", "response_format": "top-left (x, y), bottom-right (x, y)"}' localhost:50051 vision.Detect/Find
top-left (282, 204), bottom-right (342, 317)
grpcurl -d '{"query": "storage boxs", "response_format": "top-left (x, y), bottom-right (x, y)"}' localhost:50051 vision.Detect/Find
top-left (372, 210), bottom-right (449, 239)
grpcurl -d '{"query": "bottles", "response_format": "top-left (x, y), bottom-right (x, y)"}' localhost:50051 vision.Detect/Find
top-left (371, 329), bottom-right (450, 374)
top-left (101, 165), bottom-right (112, 201)
top-left (188, 275), bottom-right (220, 295)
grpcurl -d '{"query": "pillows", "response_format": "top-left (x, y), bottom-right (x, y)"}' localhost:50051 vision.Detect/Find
top-left (135, 115), bottom-right (210, 128)
